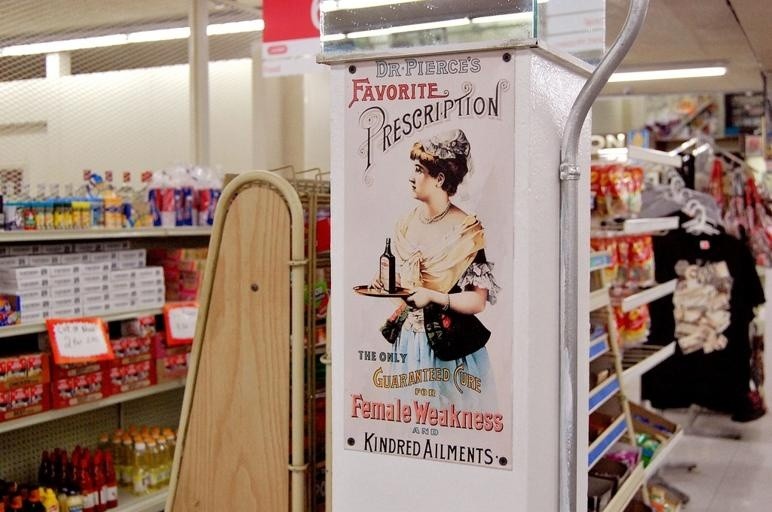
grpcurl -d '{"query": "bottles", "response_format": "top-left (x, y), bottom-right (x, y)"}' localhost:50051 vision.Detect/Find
top-left (380, 238), bottom-right (396, 293)
top-left (38, 424), bottom-right (178, 512)
top-left (105, 170), bottom-right (155, 218)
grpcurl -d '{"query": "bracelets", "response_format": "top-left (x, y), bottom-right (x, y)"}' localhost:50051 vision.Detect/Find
top-left (442, 294), bottom-right (451, 312)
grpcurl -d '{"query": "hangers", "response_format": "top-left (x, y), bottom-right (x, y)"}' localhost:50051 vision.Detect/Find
top-left (657, 166), bottom-right (719, 238)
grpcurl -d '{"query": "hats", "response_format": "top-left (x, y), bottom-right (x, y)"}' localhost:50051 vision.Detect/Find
top-left (421, 129), bottom-right (470, 161)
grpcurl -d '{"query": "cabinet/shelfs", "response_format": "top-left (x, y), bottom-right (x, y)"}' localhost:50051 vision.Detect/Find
top-left (0, 223), bottom-right (213, 512)
top-left (586, 144), bottom-right (683, 510)
top-left (161, 163), bottom-right (331, 512)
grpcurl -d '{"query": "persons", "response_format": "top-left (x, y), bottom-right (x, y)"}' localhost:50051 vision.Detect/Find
top-left (367, 128), bottom-right (499, 402)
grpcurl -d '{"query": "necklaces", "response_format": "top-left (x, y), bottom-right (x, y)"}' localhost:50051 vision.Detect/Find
top-left (418, 202), bottom-right (453, 224)
